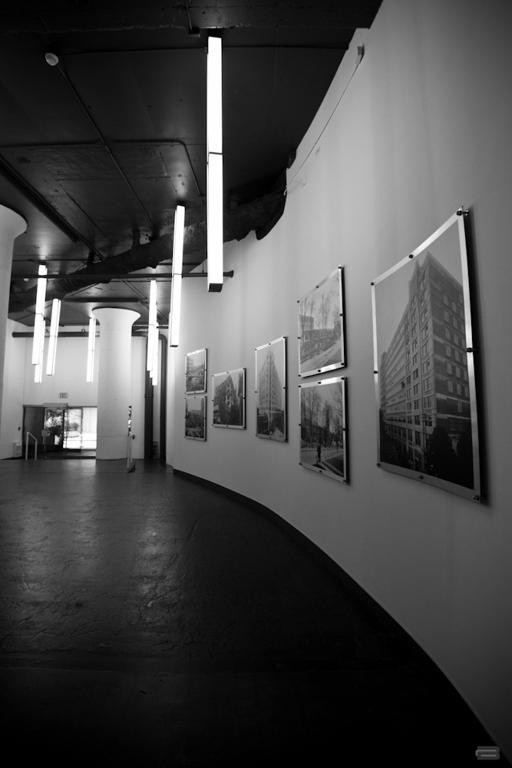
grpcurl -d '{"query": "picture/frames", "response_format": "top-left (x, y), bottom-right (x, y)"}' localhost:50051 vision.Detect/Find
top-left (183, 395), bottom-right (207, 441)
top-left (210, 366), bottom-right (247, 429)
top-left (367, 203), bottom-right (485, 506)
top-left (254, 335), bottom-right (289, 442)
top-left (183, 347), bottom-right (207, 394)
top-left (295, 264), bottom-right (347, 378)
top-left (297, 375), bottom-right (351, 485)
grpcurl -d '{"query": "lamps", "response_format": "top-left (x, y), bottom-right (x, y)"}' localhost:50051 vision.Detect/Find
top-left (30, 202), bottom-right (187, 386)
top-left (203, 33), bottom-right (226, 294)
